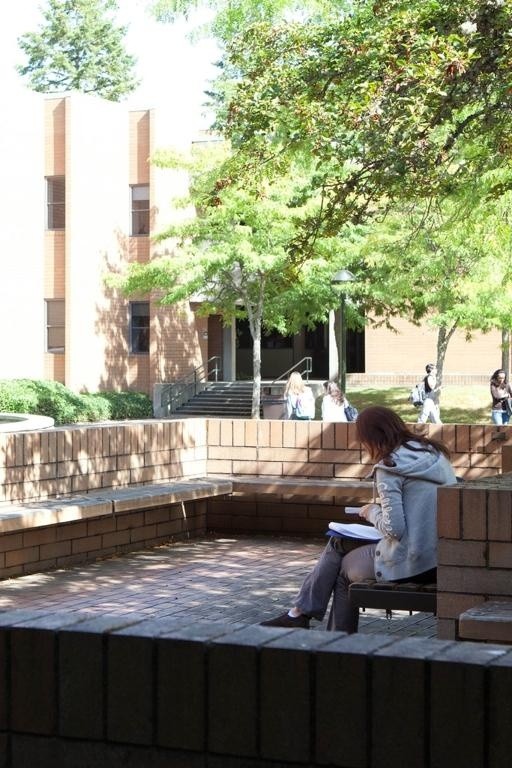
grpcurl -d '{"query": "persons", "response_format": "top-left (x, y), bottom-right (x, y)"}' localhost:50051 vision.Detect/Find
top-left (320, 381), bottom-right (350, 423)
top-left (283, 371), bottom-right (315, 421)
top-left (417, 364), bottom-right (443, 424)
top-left (260, 406), bottom-right (459, 634)
top-left (490, 369), bottom-right (512, 426)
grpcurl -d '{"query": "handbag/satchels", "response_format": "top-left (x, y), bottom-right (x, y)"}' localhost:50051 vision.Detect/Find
top-left (344, 407), bottom-right (358, 421)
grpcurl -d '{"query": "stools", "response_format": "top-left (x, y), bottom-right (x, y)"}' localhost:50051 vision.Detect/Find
top-left (347, 577), bottom-right (434, 634)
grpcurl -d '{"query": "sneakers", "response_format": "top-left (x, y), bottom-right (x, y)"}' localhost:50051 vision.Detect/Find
top-left (260, 610), bottom-right (310, 630)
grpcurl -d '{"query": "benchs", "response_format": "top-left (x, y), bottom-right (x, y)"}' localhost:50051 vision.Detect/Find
top-left (1, 473), bottom-right (380, 538)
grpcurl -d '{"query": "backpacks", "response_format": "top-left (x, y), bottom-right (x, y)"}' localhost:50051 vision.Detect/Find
top-left (295, 387), bottom-right (315, 419)
top-left (409, 381), bottom-right (426, 407)
